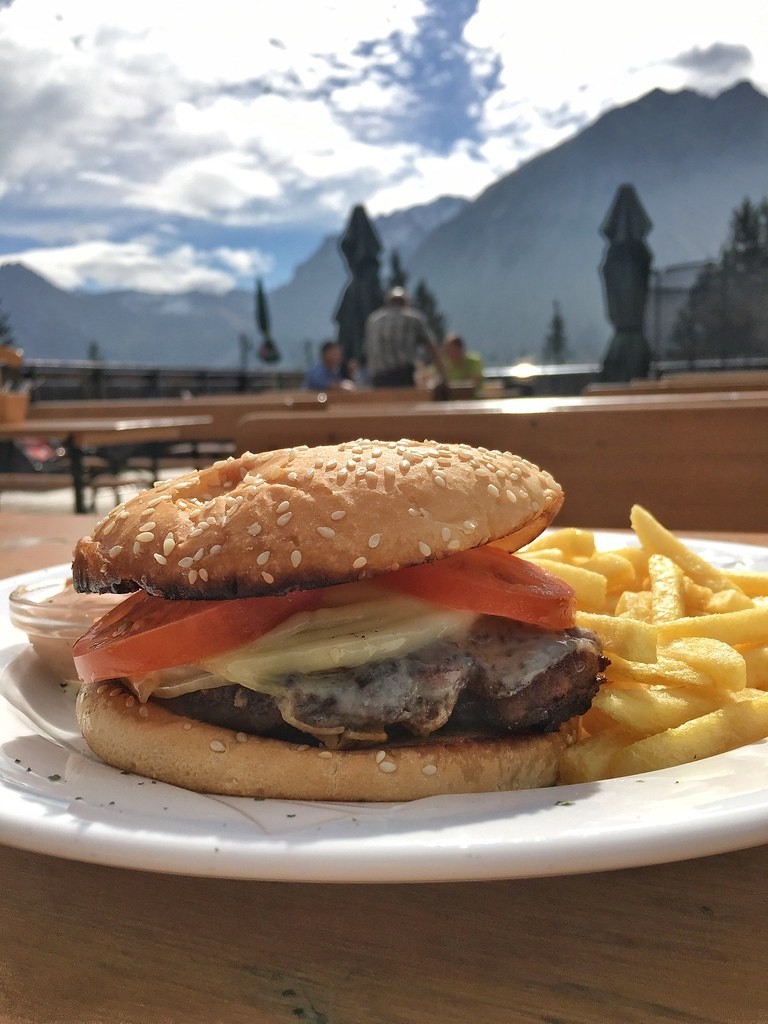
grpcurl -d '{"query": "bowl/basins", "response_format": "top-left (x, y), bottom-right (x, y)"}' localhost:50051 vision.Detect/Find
top-left (7, 577), bottom-right (137, 681)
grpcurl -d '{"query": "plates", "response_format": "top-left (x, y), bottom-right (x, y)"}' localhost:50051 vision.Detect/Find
top-left (0, 531), bottom-right (768, 885)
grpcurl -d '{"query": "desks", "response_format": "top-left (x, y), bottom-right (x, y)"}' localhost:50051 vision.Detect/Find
top-left (0, 414), bottom-right (214, 515)
top-left (0, 516), bottom-right (768, 1024)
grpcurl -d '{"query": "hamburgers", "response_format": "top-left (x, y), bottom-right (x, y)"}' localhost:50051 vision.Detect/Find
top-left (62, 438), bottom-right (613, 802)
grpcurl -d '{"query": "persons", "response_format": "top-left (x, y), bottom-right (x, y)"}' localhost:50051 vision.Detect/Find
top-left (304, 342), bottom-right (355, 393)
top-left (415, 330), bottom-right (484, 398)
top-left (361, 284), bottom-right (449, 389)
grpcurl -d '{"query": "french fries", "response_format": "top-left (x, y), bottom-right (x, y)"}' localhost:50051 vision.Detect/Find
top-left (512, 499), bottom-right (768, 784)
